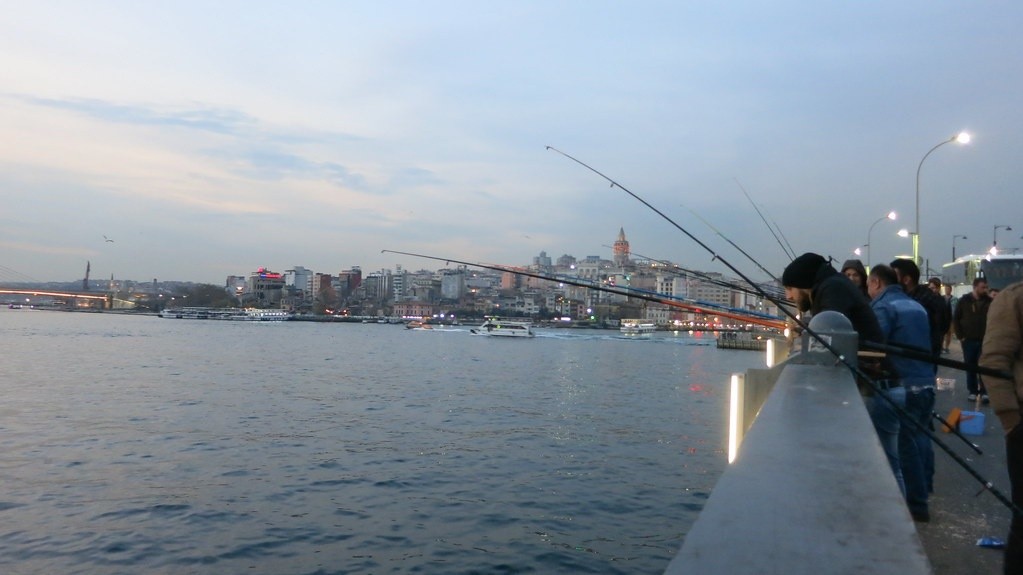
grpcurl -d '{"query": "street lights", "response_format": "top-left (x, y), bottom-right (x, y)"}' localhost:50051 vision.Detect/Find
top-left (897, 132), bottom-right (971, 268)
top-left (953, 235), bottom-right (968, 263)
top-left (994, 225), bottom-right (1012, 248)
top-left (854, 212), bottom-right (896, 276)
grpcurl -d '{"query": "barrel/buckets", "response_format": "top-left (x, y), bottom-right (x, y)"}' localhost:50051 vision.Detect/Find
top-left (959, 411), bottom-right (985, 435)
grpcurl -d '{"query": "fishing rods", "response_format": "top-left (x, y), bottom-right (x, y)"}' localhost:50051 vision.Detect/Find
top-left (734, 177), bottom-right (793, 262)
top-left (381, 246), bottom-right (1014, 384)
top-left (546, 145), bottom-right (1021, 518)
top-left (773, 223), bottom-right (798, 258)
top-left (680, 202), bottom-right (783, 287)
top-left (536, 268), bottom-right (789, 332)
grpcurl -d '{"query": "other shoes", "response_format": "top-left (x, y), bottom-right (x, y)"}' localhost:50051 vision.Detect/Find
top-left (942, 347), bottom-right (949, 354)
top-left (969, 394), bottom-right (976, 401)
top-left (912, 513), bottom-right (930, 524)
top-left (981, 394), bottom-right (989, 402)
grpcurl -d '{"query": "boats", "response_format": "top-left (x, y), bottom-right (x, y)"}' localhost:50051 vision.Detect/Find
top-left (620, 318), bottom-right (656, 333)
top-left (8, 303), bottom-right (23, 308)
top-left (157, 306), bottom-right (291, 321)
top-left (470, 315), bottom-right (535, 338)
top-left (406, 321), bottom-right (432, 329)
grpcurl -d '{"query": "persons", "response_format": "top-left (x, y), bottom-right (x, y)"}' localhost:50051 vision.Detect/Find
top-left (979, 281), bottom-right (1023, 575)
top-left (783, 253), bottom-right (1000, 522)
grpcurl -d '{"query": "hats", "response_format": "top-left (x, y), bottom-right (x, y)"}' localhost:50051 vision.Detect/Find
top-left (782, 252), bottom-right (826, 288)
